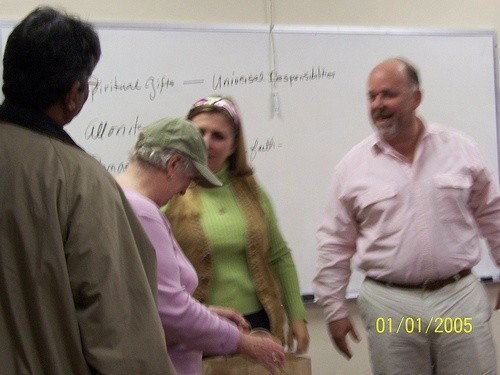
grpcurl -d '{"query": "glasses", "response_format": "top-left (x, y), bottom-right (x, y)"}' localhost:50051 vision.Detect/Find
top-left (88, 76), bottom-right (100, 96)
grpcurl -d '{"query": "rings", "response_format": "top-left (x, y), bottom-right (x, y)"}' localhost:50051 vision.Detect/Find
top-left (274, 355), bottom-right (279, 363)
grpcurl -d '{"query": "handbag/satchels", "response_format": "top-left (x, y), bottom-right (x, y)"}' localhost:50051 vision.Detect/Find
top-left (201, 331), bottom-right (312, 375)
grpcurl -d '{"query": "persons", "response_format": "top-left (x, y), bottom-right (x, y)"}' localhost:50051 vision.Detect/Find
top-left (313, 57), bottom-right (500, 375)
top-left (0, 3), bottom-right (178, 375)
top-left (159, 94), bottom-right (310, 355)
top-left (116, 115), bottom-right (287, 375)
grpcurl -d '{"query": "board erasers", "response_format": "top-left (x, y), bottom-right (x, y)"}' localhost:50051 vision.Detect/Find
top-left (301, 294), bottom-right (314, 302)
top-left (480, 277), bottom-right (493, 283)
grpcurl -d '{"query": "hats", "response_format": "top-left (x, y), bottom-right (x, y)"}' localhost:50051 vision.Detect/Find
top-left (143, 118), bottom-right (223, 190)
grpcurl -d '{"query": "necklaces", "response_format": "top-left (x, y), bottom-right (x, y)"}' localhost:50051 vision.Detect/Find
top-left (210, 187), bottom-right (226, 214)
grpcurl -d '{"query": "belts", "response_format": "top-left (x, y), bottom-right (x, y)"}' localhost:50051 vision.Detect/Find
top-left (366, 268), bottom-right (472, 291)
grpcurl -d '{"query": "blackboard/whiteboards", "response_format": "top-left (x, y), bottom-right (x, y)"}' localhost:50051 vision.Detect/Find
top-left (0, 21), bottom-right (500, 303)
